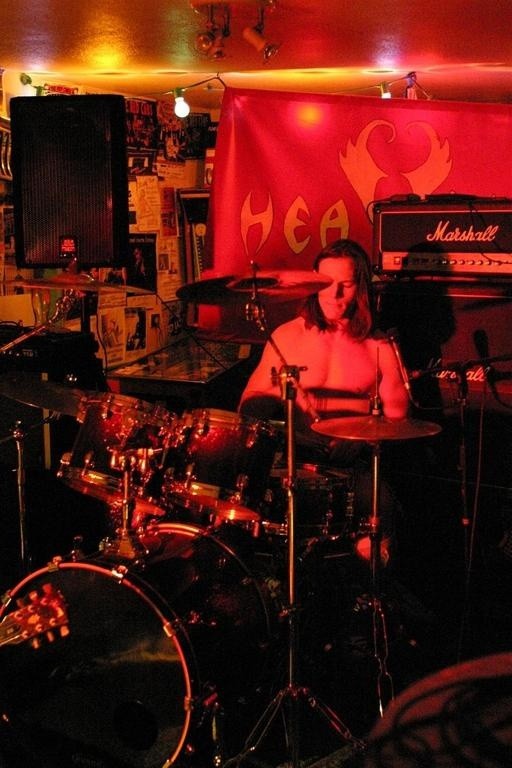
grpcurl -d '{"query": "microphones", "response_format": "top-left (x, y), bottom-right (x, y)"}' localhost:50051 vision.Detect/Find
top-left (48, 287), bottom-right (77, 328)
top-left (472, 328), bottom-right (501, 401)
top-left (387, 327), bottom-right (410, 390)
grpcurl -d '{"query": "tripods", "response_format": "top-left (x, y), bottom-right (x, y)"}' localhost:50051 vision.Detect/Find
top-left (323, 383), bottom-right (489, 738)
top-left (0, 309), bottom-right (71, 577)
top-left (219, 364), bottom-right (370, 768)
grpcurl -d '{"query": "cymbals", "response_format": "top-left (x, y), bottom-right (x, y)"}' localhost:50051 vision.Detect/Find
top-left (313, 415), bottom-right (440, 441)
top-left (5, 275), bottom-right (153, 296)
top-left (175, 270), bottom-right (332, 306)
top-left (2, 378), bottom-right (78, 418)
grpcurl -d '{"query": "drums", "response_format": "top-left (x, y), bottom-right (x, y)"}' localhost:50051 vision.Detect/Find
top-left (61, 391), bottom-right (180, 517)
top-left (175, 407), bottom-right (280, 522)
top-left (266, 464), bottom-right (352, 536)
top-left (1, 521), bottom-right (271, 764)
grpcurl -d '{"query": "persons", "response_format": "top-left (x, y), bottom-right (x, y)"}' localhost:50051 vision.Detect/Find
top-left (237, 240), bottom-right (410, 565)
top-left (13, 269), bottom-right (25, 294)
top-left (127, 246), bottom-right (155, 291)
top-left (159, 256), bottom-right (177, 274)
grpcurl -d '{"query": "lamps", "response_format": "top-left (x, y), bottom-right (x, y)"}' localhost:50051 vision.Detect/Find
top-left (377, 76), bottom-right (420, 100)
top-left (187, 0), bottom-right (281, 67)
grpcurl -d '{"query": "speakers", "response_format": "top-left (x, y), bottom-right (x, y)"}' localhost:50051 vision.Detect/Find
top-left (8, 94), bottom-right (133, 270)
top-left (375, 277), bottom-right (511, 373)
